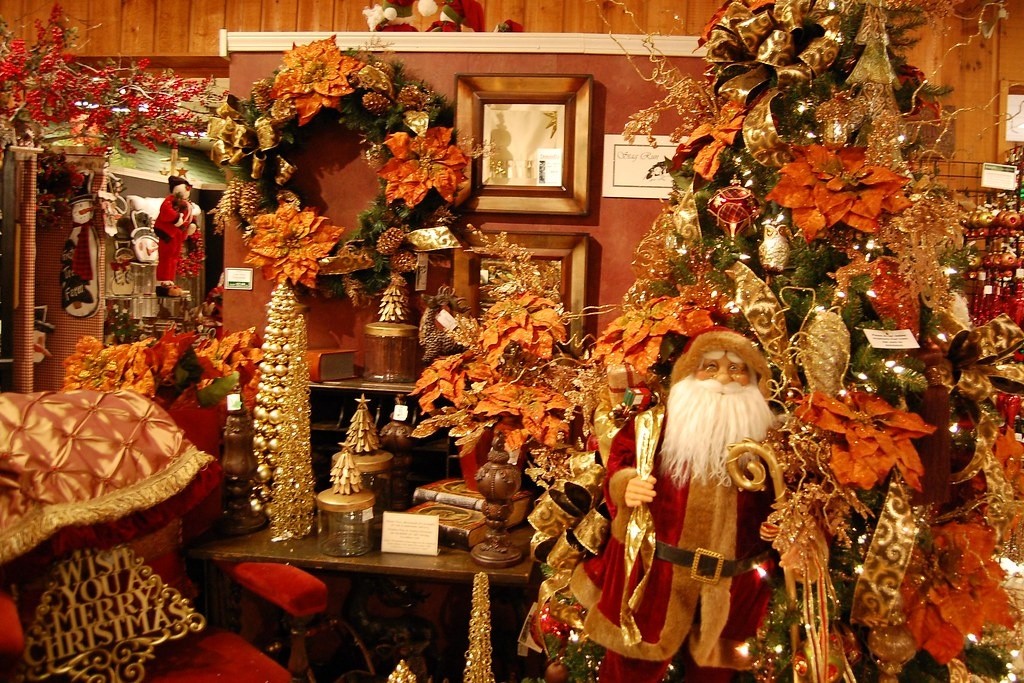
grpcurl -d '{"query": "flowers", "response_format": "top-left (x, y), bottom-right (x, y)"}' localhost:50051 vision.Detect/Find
top-left (0, 0), bottom-right (1024, 682)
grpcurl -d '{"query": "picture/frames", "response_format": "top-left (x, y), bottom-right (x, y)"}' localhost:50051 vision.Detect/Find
top-left (453, 72), bottom-right (595, 215)
top-left (453, 231), bottom-right (588, 357)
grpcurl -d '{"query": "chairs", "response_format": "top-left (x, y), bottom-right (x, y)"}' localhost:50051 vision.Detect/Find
top-left (0, 386), bottom-right (327, 681)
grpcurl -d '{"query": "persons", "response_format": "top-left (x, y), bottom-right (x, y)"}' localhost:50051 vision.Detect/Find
top-left (153, 175), bottom-right (196, 299)
top-left (571, 327), bottom-right (788, 683)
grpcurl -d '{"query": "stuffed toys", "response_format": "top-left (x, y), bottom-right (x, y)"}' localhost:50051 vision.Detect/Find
top-left (361, 0), bottom-right (524, 33)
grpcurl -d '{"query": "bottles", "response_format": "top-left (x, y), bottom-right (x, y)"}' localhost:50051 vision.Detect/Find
top-left (332, 450), bottom-right (393, 525)
top-left (363, 322), bottom-right (419, 383)
top-left (315, 486), bottom-right (377, 557)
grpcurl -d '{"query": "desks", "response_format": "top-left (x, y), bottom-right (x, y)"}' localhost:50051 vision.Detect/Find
top-left (184, 519), bottom-right (536, 585)
